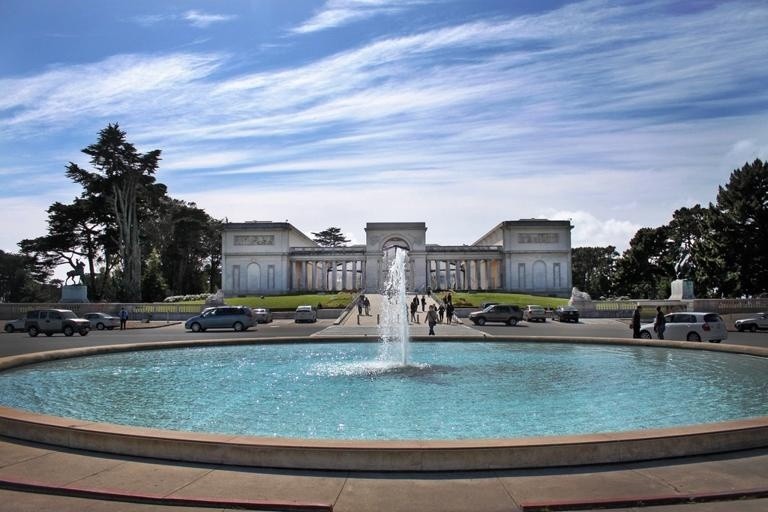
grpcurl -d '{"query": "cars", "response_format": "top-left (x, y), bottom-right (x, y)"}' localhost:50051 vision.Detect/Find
top-left (553, 304), bottom-right (579, 322)
top-left (81, 312), bottom-right (121, 330)
top-left (5, 318), bottom-right (25, 333)
top-left (521, 304), bottom-right (547, 322)
top-left (254, 308), bottom-right (273, 323)
top-left (735, 312), bottom-right (768, 332)
top-left (295, 305), bottom-right (317, 322)
top-left (481, 301), bottom-right (499, 309)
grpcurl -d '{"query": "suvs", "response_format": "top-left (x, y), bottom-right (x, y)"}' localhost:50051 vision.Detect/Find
top-left (470, 304), bottom-right (524, 327)
top-left (25, 309), bottom-right (87, 337)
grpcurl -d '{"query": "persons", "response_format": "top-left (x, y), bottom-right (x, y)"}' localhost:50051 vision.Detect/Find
top-left (410, 293), bottom-right (454, 335)
top-left (118, 308), bottom-right (128, 330)
top-left (654, 306), bottom-right (666, 339)
top-left (356, 294), bottom-right (370, 316)
top-left (632, 306), bottom-right (643, 338)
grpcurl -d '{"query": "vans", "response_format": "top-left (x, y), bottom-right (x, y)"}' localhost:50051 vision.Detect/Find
top-left (639, 311), bottom-right (729, 343)
top-left (185, 306), bottom-right (257, 333)
